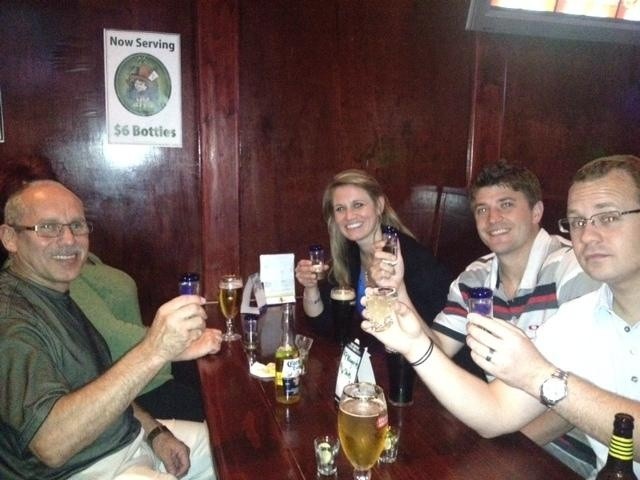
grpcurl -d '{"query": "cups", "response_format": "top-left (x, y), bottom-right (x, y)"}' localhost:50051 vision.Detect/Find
top-left (178, 273), bottom-right (200, 296)
top-left (380, 426), bottom-right (399, 463)
top-left (308, 245), bottom-right (326, 281)
top-left (370, 286), bottom-right (397, 330)
top-left (382, 225), bottom-right (398, 257)
top-left (294, 334), bottom-right (313, 361)
top-left (465, 289), bottom-right (493, 318)
top-left (314, 435), bottom-right (339, 475)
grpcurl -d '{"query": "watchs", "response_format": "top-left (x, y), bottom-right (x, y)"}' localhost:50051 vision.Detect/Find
top-left (536, 366), bottom-right (571, 408)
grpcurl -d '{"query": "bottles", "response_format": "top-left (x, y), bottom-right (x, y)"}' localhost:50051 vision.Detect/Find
top-left (386, 346), bottom-right (416, 406)
top-left (594, 413), bottom-right (638, 480)
top-left (274, 304), bottom-right (305, 403)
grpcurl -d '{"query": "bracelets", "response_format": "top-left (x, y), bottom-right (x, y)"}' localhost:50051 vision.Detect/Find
top-left (409, 339), bottom-right (435, 366)
top-left (144, 424), bottom-right (174, 449)
top-left (301, 291), bottom-right (323, 306)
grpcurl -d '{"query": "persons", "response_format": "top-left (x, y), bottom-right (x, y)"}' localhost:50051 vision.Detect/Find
top-left (1, 180), bottom-right (219, 478)
top-left (359, 151), bottom-right (640, 480)
top-left (1, 150), bottom-right (224, 423)
top-left (294, 167), bottom-right (447, 348)
top-left (365, 159), bottom-right (602, 383)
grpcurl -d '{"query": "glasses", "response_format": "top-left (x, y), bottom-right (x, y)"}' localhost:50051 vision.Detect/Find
top-left (558, 208), bottom-right (640, 234)
top-left (9, 221), bottom-right (93, 238)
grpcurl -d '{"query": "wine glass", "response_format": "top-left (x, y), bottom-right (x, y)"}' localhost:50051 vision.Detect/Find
top-left (338, 382), bottom-right (390, 479)
top-left (330, 286), bottom-right (357, 356)
top-left (236, 319), bottom-right (260, 376)
top-left (219, 274), bottom-right (243, 341)
